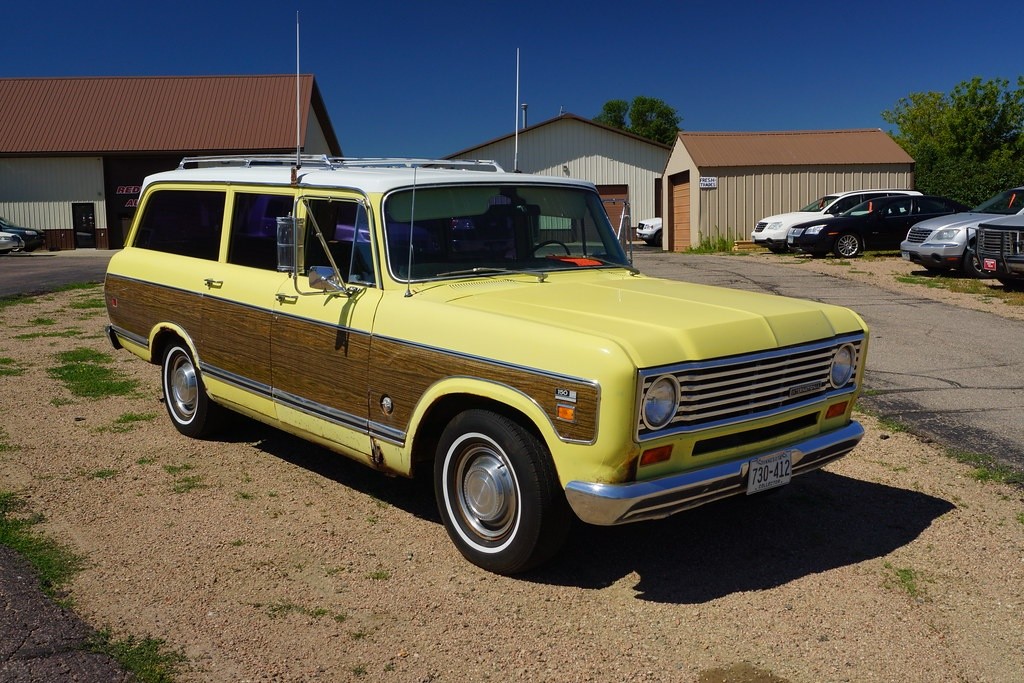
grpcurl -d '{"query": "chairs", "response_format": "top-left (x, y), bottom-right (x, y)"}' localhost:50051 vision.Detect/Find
top-left (889, 205), bottom-right (902, 216)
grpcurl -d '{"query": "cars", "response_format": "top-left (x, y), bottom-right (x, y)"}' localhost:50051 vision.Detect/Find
top-left (0, 232), bottom-right (26, 255)
top-left (785, 194), bottom-right (974, 259)
top-left (637, 216), bottom-right (663, 246)
top-left (750, 187), bottom-right (922, 255)
top-left (899, 187), bottom-right (1024, 279)
top-left (965, 209), bottom-right (1024, 287)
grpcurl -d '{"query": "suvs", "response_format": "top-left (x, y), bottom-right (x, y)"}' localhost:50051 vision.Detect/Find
top-left (0, 216), bottom-right (47, 252)
top-left (97, 152), bottom-right (874, 576)
